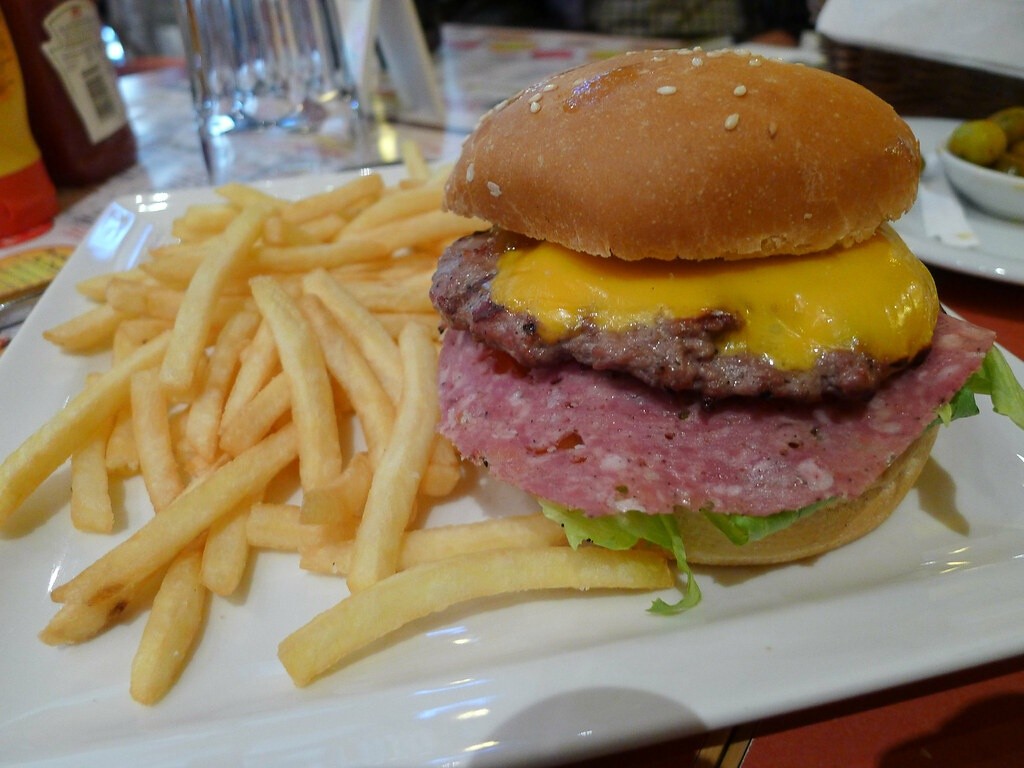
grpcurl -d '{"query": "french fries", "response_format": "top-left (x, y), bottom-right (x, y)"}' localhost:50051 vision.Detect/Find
top-left (0, 137), bottom-right (673, 704)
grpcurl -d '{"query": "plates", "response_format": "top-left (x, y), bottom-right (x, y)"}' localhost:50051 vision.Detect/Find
top-left (883, 115), bottom-right (1024, 285)
top-left (0, 152), bottom-right (1024, 768)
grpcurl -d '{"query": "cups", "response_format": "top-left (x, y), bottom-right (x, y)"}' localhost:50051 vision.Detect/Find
top-left (172, 0), bottom-right (384, 186)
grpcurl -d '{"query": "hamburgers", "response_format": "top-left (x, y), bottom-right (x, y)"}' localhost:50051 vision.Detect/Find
top-left (427, 47), bottom-right (1024, 621)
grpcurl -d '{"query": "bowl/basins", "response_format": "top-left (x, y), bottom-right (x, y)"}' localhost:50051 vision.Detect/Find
top-left (936, 132), bottom-right (1024, 221)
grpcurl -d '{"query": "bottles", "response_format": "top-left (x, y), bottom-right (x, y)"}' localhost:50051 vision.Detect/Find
top-left (0, 0), bottom-right (139, 246)
